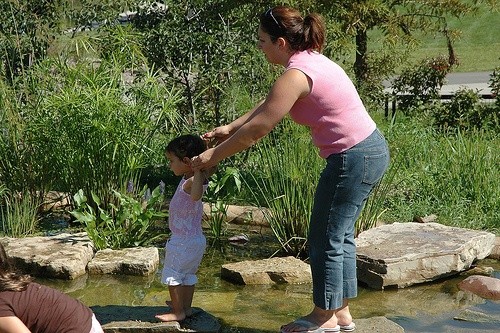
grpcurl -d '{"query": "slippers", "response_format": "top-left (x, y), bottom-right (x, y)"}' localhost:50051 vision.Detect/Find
top-left (340, 321), bottom-right (356, 332)
top-left (280, 318), bottom-right (340, 333)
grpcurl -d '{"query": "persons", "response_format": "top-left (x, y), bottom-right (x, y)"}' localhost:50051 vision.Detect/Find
top-left (194, 5), bottom-right (390, 332)
top-left (0, 270), bottom-right (107, 333)
top-left (154, 133), bottom-right (216, 321)
